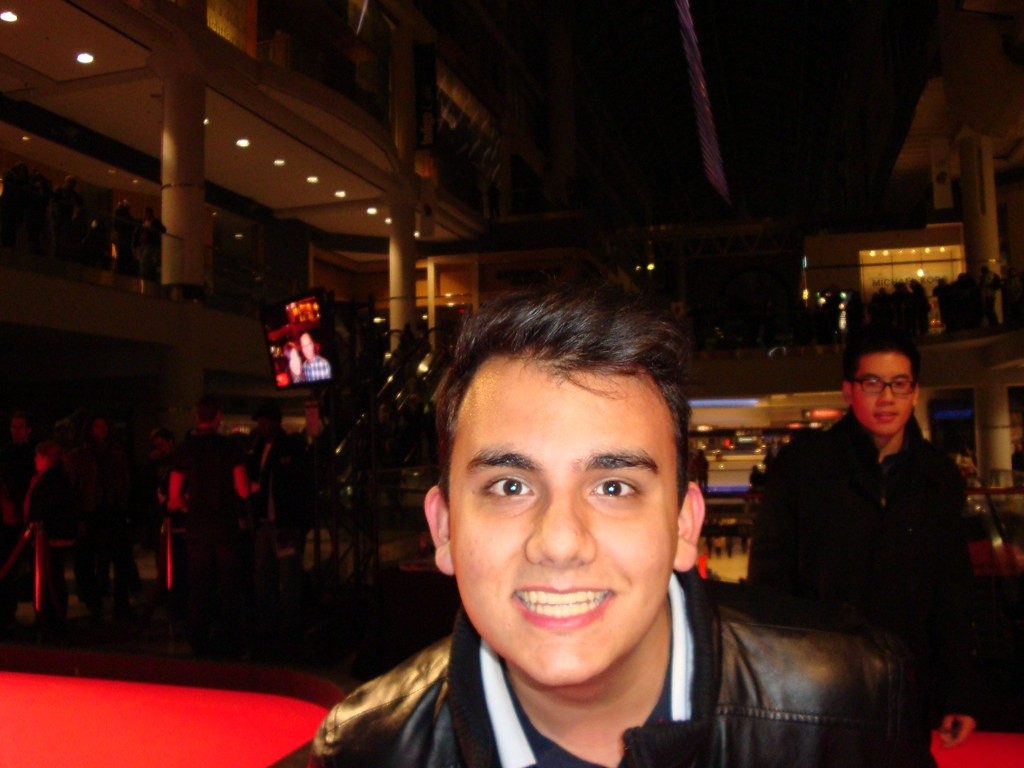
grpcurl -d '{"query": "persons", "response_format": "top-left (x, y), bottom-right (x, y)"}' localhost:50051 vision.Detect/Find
top-left (751, 320), bottom-right (990, 768)
top-left (307, 300), bottom-right (896, 768)
top-left (0, 161), bottom-right (1024, 686)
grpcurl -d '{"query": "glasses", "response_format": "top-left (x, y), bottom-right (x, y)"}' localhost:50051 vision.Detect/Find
top-left (850, 378), bottom-right (916, 396)
